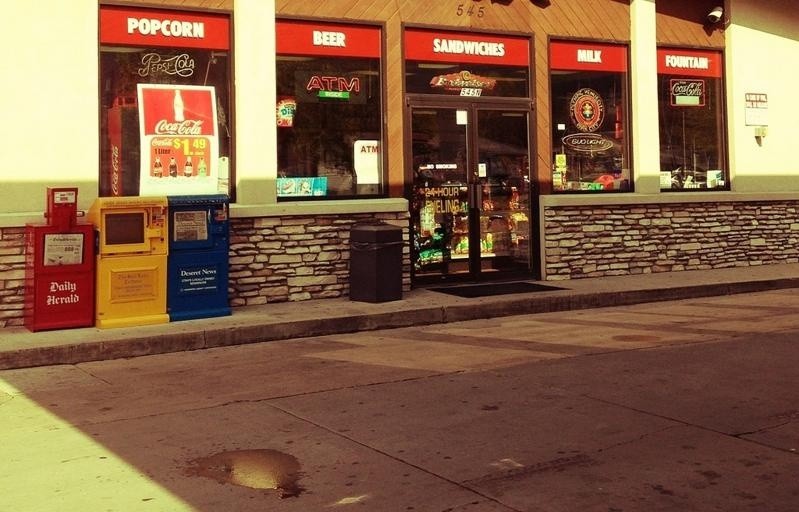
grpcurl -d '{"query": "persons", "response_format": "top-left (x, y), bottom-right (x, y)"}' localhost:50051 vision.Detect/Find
top-left (590, 156), bottom-right (616, 192)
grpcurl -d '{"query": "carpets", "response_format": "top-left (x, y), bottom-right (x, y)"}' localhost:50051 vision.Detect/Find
top-left (425, 280), bottom-right (570, 300)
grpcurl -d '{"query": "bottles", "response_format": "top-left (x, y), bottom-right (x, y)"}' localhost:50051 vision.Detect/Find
top-left (198, 157), bottom-right (207, 177)
top-left (167, 157), bottom-right (178, 178)
top-left (153, 156), bottom-right (164, 178)
top-left (184, 157), bottom-right (194, 178)
